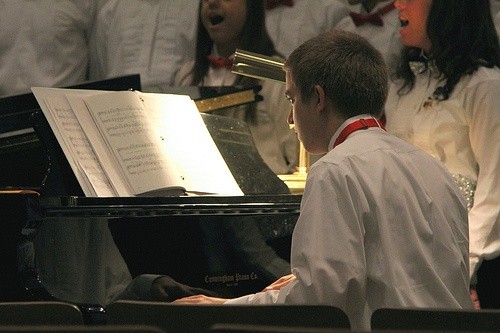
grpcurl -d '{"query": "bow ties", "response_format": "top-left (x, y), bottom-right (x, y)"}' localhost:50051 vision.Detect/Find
top-left (206, 55), bottom-right (242, 77)
top-left (265, 0), bottom-right (293, 10)
top-left (348, 10), bottom-right (383, 27)
top-left (408, 59), bottom-right (440, 78)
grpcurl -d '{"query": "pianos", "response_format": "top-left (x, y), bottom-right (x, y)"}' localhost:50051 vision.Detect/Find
top-left (0, 74), bottom-right (303, 325)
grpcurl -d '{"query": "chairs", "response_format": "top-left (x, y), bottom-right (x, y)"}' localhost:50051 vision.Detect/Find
top-left (0, 302), bottom-right (500, 333)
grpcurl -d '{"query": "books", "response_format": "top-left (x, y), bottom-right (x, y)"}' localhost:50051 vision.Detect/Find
top-left (31, 87), bottom-right (246, 198)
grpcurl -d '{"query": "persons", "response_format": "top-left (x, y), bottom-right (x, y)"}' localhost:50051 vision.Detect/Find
top-left (0, 0), bottom-right (500, 175)
top-left (382, 0), bottom-right (500, 310)
top-left (17, 215), bottom-right (225, 302)
top-left (174, 29), bottom-right (473, 331)
